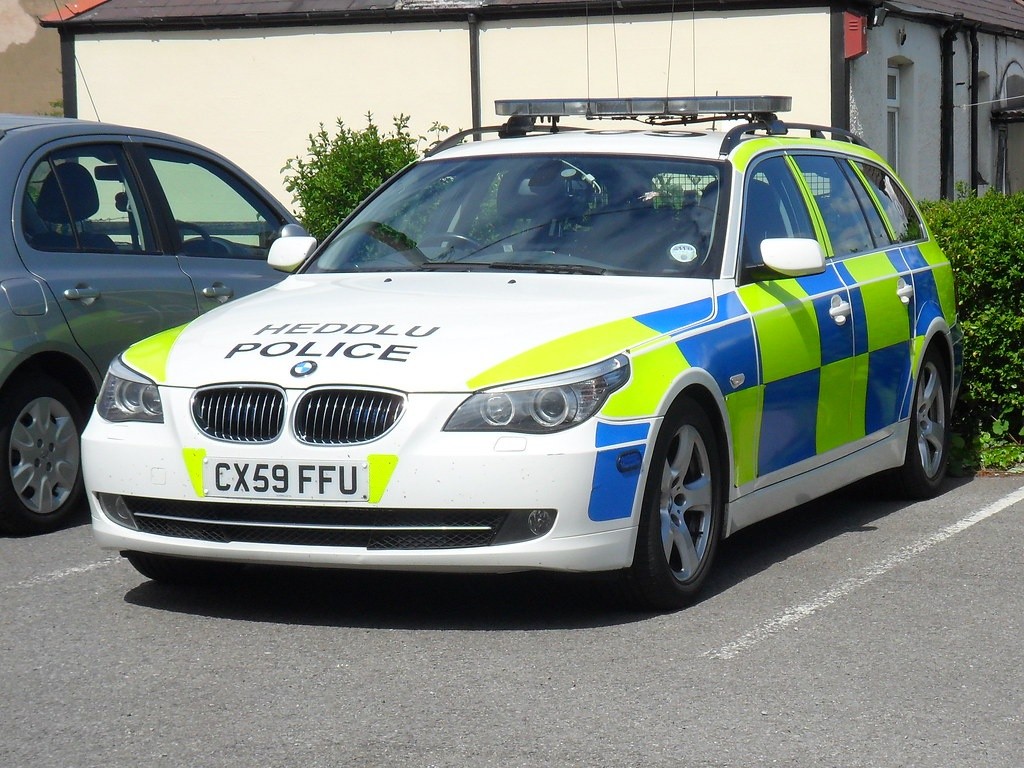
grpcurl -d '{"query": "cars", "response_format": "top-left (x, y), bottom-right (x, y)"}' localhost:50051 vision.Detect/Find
top-left (1, 113), bottom-right (317, 538)
top-left (512, 90), bottom-right (514, 98)
top-left (76, 93), bottom-right (963, 619)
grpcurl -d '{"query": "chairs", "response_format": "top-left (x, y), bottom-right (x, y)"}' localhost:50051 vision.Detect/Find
top-left (37, 162), bottom-right (121, 253)
top-left (490, 163), bottom-right (572, 256)
top-left (700, 180), bottom-right (784, 271)
